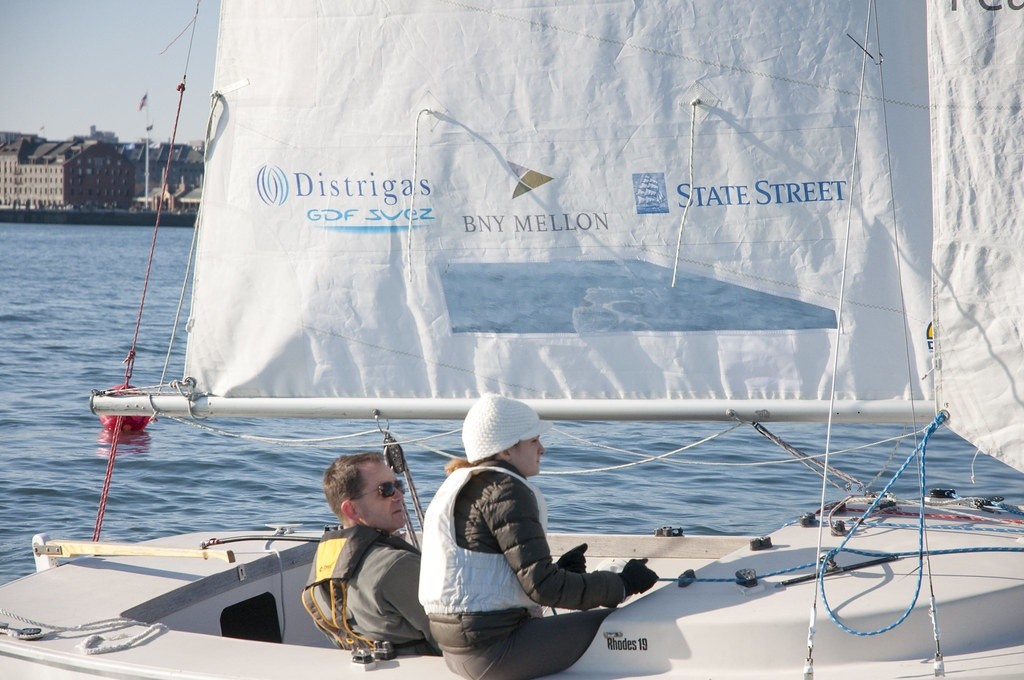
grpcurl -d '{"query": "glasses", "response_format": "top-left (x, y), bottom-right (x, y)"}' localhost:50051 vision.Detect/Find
top-left (348, 479), bottom-right (405, 501)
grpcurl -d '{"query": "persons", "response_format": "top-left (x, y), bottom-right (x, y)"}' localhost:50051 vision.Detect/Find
top-left (417, 394), bottom-right (663, 680)
top-left (301, 452), bottom-right (443, 658)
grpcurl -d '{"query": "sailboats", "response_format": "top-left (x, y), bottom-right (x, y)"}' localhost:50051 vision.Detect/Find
top-left (0, 0), bottom-right (1024, 680)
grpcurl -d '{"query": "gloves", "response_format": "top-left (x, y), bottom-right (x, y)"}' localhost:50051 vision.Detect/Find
top-left (620, 557), bottom-right (660, 596)
top-left (554, 542), bottom-right (587, 574)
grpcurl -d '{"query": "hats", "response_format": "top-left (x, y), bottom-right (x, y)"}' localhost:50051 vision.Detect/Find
top-left (460, 392), bottom-right (554, 463)
